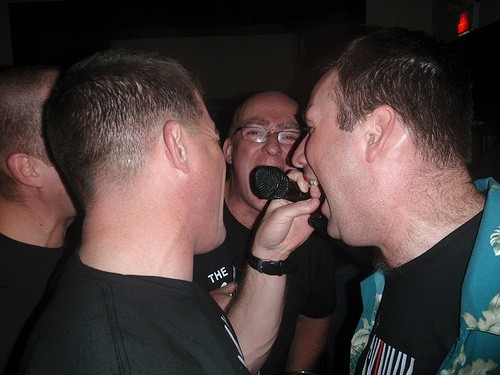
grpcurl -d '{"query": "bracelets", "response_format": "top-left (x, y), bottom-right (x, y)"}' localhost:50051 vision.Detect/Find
top-left (248, 254), bottom-right (293, 276)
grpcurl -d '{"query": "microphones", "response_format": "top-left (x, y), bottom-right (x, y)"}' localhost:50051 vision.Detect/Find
top-left (254, 166), bottom-right (324, 214)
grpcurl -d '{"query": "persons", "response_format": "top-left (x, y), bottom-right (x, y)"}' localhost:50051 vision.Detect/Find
top-left (17, 48), bottom-right (320, 374)
top-left (0, 66), bottom-right (77, 375)
top-left (193, 91), bottom-right (337, 374)
top-left (292, 27), bottom-right (500, 375)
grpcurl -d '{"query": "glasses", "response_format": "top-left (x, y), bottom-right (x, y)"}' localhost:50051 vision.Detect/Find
top-left (231, 123), bottom-right (304, 145)
